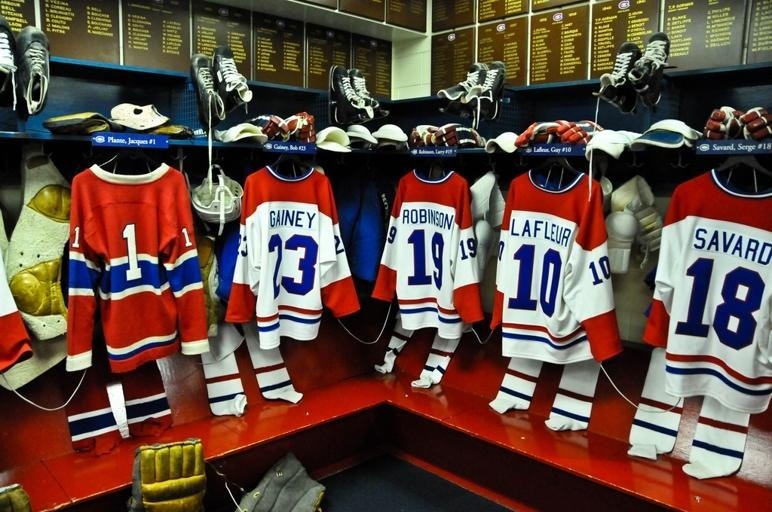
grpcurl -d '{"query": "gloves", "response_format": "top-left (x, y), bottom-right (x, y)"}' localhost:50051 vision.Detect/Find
top-left (428, 123), bottom-right (486, 148)
top-left (285, 112), bottom-right (317, 143)
top-left (245, 115), bottom-right (290, 141)
top-left (704, 106), bottom-right (743, 140)
top-left (516, 121), bottom-right (568, 147)
top-left (548, 120), bottom-right (603, 145)
top-left (408, 125), bottom-right (439, 148)
top-left (740, 106), bottom-right (772, 140)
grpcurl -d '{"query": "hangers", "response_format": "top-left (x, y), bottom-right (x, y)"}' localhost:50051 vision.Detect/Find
top-left (534, 155), bottom-right (578, 177)
top-left (265, 150), bottom-right (319, 181)
top-left (409, 154), bottom-right (456, 185)
top-left (714, 152), bottom-right (772, 181)
top-left (93, 148), bottom-right (162, 179)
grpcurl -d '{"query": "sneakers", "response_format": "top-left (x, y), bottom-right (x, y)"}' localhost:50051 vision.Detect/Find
top-left (16, 26), bottom-right (50, 115)
top-left (0, 15), bottom-right (17, 112)
top-left (211, 46), bottom-right (252, 114)
top-left (437, 63), bottom-right (489, 120)
top-left (593, 42), bottom-right (642, 118)
top-left (461, 61), bottom-right (505, 123)
top-left (628, 32), bottom-right (670, 108)
top-left (349, 68), bottom-right (389, 121)
top-left (190, 54), bottom-right (227, 127)
top-left (331, 65), bottom-right (374, 125)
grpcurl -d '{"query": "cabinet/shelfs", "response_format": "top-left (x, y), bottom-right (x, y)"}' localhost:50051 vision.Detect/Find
top-left (0, 1), bottom-right (771, 512)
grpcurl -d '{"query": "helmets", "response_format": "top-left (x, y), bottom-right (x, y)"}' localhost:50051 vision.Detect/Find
top-left (190, 176), bottom-right (244, 224)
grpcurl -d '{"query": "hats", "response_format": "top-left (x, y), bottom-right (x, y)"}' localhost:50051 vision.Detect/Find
top-left (630, 119), bottom-right (703, 152)
top-left (585, 130), bottom-right (641, 161)
top-left (484, 131), bottom-right (519, 153)
top-left (215, 123), bottom-right (268, 145)
top-left (316, 127), bottom-right (352, 153)
top-left (346, 125), bottom-right (378, 145)
top-left (372, 124), bottom-right (408, 141)
